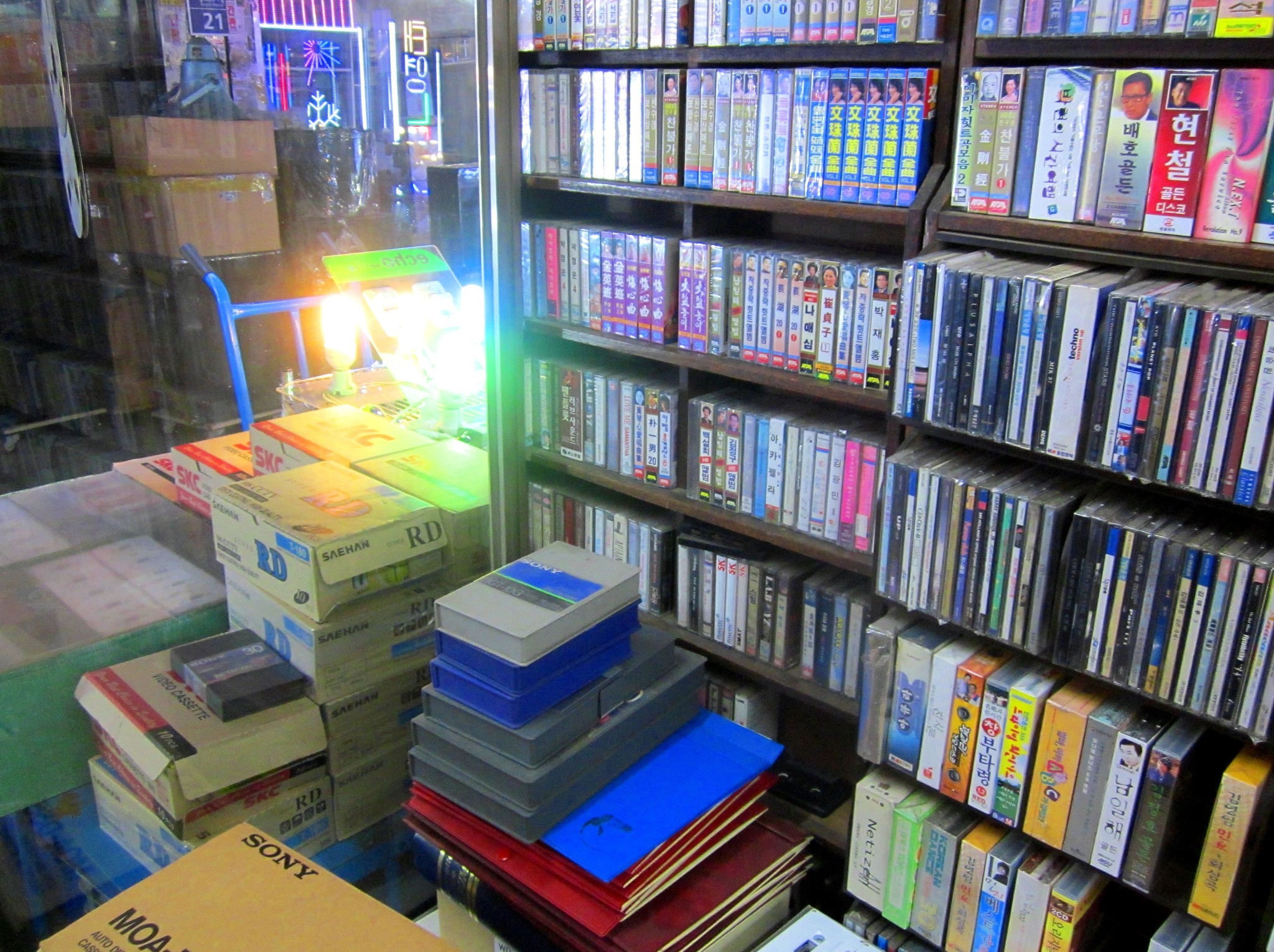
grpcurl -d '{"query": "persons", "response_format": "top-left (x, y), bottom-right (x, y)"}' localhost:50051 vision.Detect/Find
top-left (634, 389), bottom-right (644, 406)
top-left (980, 74), bottom-right (1017, 102)
top-left (1118, 738), bottom-right (1142, 772)
top-left (665, 77), bottom-right (677, 96)
top-left (644, 73), bottom-right (656, 95)
top-left (1146, 757), bottom-right (1175, 787)
top-left (646, 393), bottom-right (658, 409)
top-left (728, 414), bottom-right (739, 431)
top-left (601, 239), bottom-right (650, 264)
top-left (764, 74), bottom-right (791, 95)
top-left (701, 406), bottom-right (711, 424)
top-left (731, 253), bottom-right (902, 295)
top-left (1122, 72), bottom-right (1157, 121)
top-left (955, 674), bottom-right (979, 706)
top-left (993, 862), bottom-right (1009, 886)
top-left (687, 72), bottom-right (757, 98)
top-left (717, 410), bottom-right (726, 427)
top-left (812, 78), bottom-right (924, 105)
top-left (1168, 76), bottom-right (1200, 109)
top-left (660, 395), bottom-right (670, 413)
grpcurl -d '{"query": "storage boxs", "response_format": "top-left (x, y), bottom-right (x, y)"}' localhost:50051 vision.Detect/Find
top-left (0, 17), bottom-right (492, 952)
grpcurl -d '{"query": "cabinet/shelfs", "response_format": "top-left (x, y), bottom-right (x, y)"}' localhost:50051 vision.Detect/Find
top-left (474, 0), bottom-right (1274, 952)
